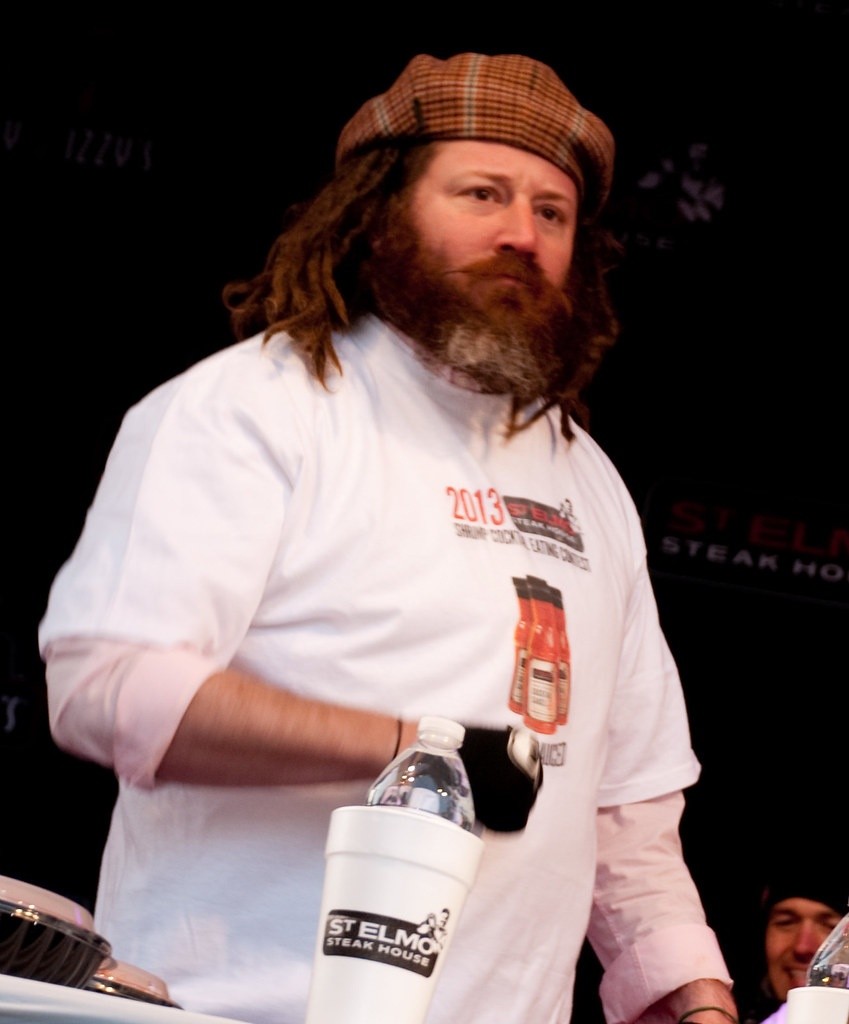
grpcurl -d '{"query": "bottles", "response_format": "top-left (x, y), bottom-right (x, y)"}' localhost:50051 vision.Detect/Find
top-left (804, 901), bottom-right (849, 989)
top-left (367, 716), bottom-right (475, 831)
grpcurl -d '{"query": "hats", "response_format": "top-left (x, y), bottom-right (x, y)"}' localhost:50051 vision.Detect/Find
top-left (764, 860), bottom-right (849, 921)
top-left (334, 52), bottom-right (614, 218)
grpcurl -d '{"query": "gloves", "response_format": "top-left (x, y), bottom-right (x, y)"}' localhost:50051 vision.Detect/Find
top-left (461, 724), bottom-right (545, 834)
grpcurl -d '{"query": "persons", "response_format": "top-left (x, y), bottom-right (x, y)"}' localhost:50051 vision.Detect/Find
top-left (38, 52), bottom-right (739, 1024)
top-left (761, 845), bottom-right (848, 1023)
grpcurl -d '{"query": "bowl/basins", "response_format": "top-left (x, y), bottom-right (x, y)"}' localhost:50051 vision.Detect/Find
top-left (0, 898), bottom-right (116, 988)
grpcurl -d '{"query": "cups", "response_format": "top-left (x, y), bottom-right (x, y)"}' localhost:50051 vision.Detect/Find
top-left (306, 804), bottom-right (484, 1024)
top-left (785, 987), bottom-right (849, 1024)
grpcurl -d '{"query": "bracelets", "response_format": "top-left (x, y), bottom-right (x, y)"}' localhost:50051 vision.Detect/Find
top-left (392, 719), bottom-right (402, 761)
top-left (677, 1005), bottom-right (738, 1024)
